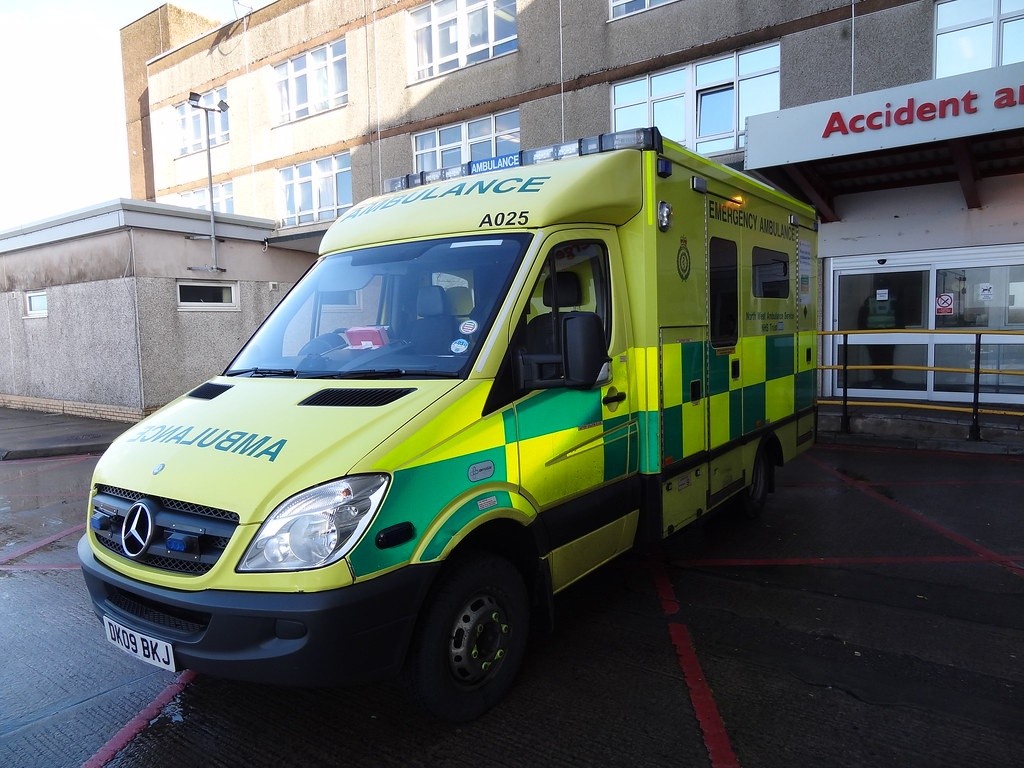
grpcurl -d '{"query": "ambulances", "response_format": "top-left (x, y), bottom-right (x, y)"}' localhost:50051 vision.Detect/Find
top-left (75, 125), bottom-right (820, 725)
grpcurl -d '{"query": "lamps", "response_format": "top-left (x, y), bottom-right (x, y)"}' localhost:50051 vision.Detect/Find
top-left (217, 99), bottom-right (229, 112)
top-left (189, 92), bottom-right (201, 102)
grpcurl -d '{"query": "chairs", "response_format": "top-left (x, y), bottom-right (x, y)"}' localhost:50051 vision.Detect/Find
top-left (401, 285), bottom-right (462, 354)
top-left (524, 271), bottom-right (581, 378)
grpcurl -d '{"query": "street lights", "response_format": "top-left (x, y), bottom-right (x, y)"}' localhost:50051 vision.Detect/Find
top-left (187, 92), bottom-right (229, 273)
